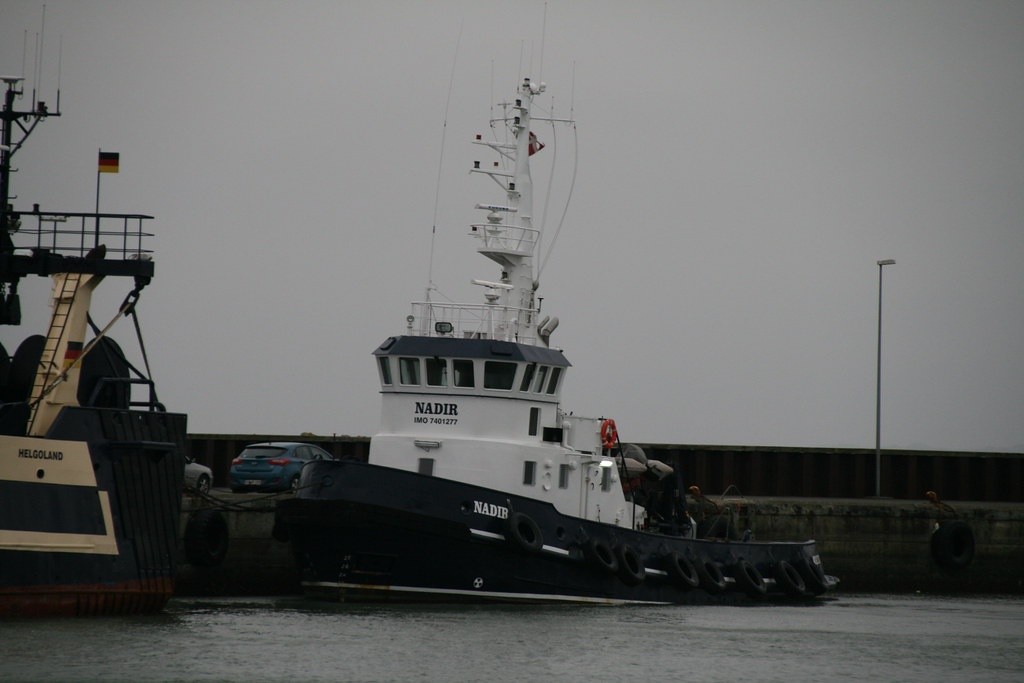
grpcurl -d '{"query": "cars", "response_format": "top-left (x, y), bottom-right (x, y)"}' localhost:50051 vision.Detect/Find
top-left (183, 455), bottom-right (214, 496)
top-left (229, 441), bottom-right (337, 495)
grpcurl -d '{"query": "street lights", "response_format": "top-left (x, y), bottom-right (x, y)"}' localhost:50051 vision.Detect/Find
top-left (875, 259), bottom-right (897, 497)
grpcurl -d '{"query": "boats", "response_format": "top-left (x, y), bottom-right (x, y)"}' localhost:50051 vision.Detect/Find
top-left (283, 67), bottom-right (844, 604)
top-left (0, 31), bottom-right (192, 617)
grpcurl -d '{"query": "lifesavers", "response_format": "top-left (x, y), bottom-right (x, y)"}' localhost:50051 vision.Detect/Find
top-left (663, 551), bottom-right (699, 591)
top-left (600, 418), bottom-right (617, 449)
top-left (502, 511), bottom-right (544, 557)
top-left (614, 543), bottom-right (646, 587)
top-left (582, 536), bottom-right (620, 579)
top-left (182, 506), bottom-right (229, 569)
top-left (695, 556), bottom-right (727, 596)
top-left (776, 559), bottom-right (807, 598)
top-left (734, 559), bottom-right (766, 600)
